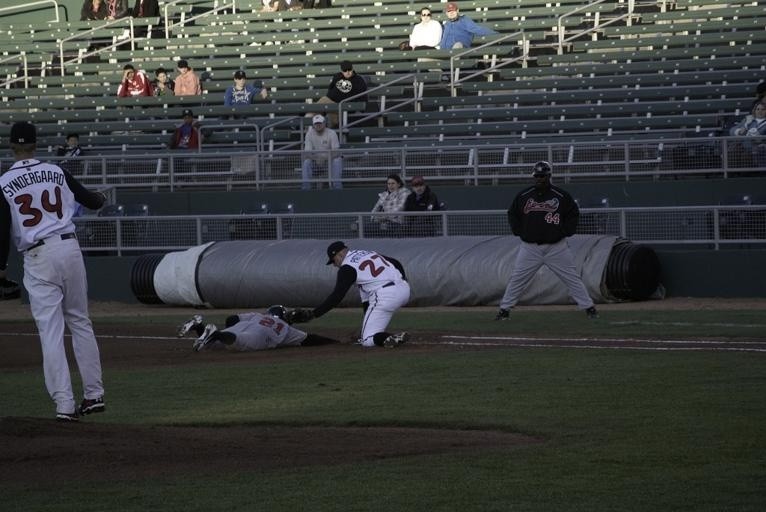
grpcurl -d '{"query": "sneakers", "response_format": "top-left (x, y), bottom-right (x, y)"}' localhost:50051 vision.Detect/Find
top-left (585, 306), bottom-right (603, 320)
top-left (383, 330), bottom-right (411, 350)
top-left (174, 313), bottom-right (204, 338)
top-left (190, 322), bottom-right (218, 353)
top-left (493, 309), bottom-right (512, 323)
top-left (78, 397), bottom-right (106, 416)
top-left (55, 407), bottom-right (79, 423)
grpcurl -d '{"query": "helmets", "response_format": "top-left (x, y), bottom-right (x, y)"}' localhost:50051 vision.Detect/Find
top-left (532, 160), bottom-right (551, 175)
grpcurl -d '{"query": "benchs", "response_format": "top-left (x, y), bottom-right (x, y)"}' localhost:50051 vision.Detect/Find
top-left (0, 0), bottom-right (766, 191)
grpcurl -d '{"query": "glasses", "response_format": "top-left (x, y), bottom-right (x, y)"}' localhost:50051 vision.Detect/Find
top-left (421, 13), bottom-right (432, 17)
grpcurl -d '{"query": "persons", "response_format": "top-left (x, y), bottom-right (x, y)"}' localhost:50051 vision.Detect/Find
top-left (495, 160), bottom-right (599, 320)
top-left (304, 59), bottom-right (368, 131)
top-left (408, 6), bottom-right (449, 82)
top-left (404, 175), bottom-right (440, 237)
top-left (58, 133), bottom-right (84, 175)
top-left (0, 118), bottom-right (109, 423)
top-left (370, 173), bottom-right (411, 238)
top-left (293, 240), bottom-right (412, 350)
top-left (301, 114), bottom-right (344, 189)
top-left (440, 3), bottom-right (500, 49)
top-left (80, 0), bottom-right (333, 150)
top-left (720, 101), bottom-right (766, 178)
top-left (176, 304), bottom-right (340, 353)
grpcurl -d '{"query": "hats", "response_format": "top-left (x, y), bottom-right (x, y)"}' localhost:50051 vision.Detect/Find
top-left (323, 239), bottom-right (347, 266)
top-left (176, 59), bottom-right (188, 68)
top-left (338, 60), bottom-right (353, 70)
top-left (266, 304), bottom-right (288, 321)
top-left (311, 114), bottom-right (325, 124)
top-left (443, 1), bottom-right (459, 13)
top-left (180, 109), bottom-right (194, 117)
top-left (232, 70), bottom-right (247, 80)
top-left (8, 120), bottom-right (37, 144)
top-left (409, 175), bottom-right (425, 186)
